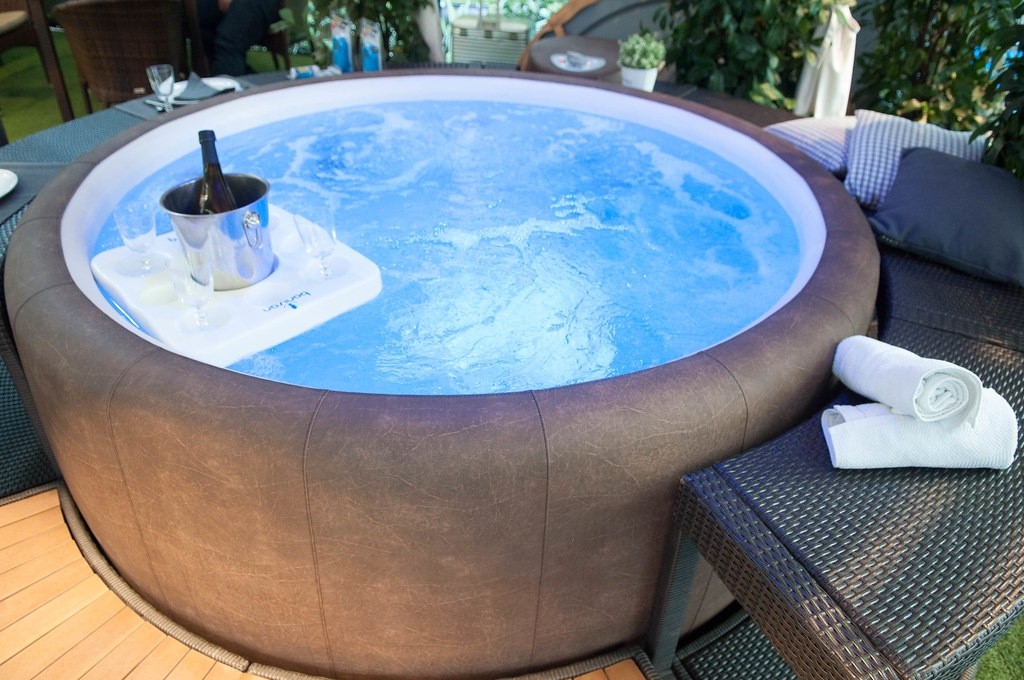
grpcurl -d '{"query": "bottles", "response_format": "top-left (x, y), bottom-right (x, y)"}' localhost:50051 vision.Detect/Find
top-left (197, 129), bottom-right (238, 216)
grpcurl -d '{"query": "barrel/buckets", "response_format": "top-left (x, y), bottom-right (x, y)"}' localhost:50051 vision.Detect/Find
top-left (158, 173), bottom-right (275, 290)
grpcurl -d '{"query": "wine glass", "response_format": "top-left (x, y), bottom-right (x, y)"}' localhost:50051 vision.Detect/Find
top-left (112, 201), bottom-right (156, 275)
top-left (145, 64), bottom-right (175, 113)
top-left (165, 251), bottom-right (215, 330)
top-left (294, 205), bottom-right (341, 281)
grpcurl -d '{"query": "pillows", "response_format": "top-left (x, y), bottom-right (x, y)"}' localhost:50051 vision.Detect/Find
top-left (763, 109), bottom-right (1024, 292)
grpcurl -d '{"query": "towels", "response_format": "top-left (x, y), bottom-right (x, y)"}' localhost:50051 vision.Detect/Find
top-left (821, 335), bottom-right (1018, 470)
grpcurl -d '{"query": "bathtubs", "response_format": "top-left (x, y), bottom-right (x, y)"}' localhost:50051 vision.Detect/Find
top-left (2, 65), bottom-right (879, 680)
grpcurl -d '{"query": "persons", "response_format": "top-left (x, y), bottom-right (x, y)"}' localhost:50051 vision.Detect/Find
top-left (195, 0), bottom-right (278, 78)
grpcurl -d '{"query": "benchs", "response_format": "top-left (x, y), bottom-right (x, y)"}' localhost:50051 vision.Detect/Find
top-left (648, 247), bottom-right (1024, 680)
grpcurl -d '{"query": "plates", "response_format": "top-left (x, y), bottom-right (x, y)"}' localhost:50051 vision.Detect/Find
top-left (0, 168), bottom-right (19, 199)
top-left (157, 78), bottom-right (241, 106)
